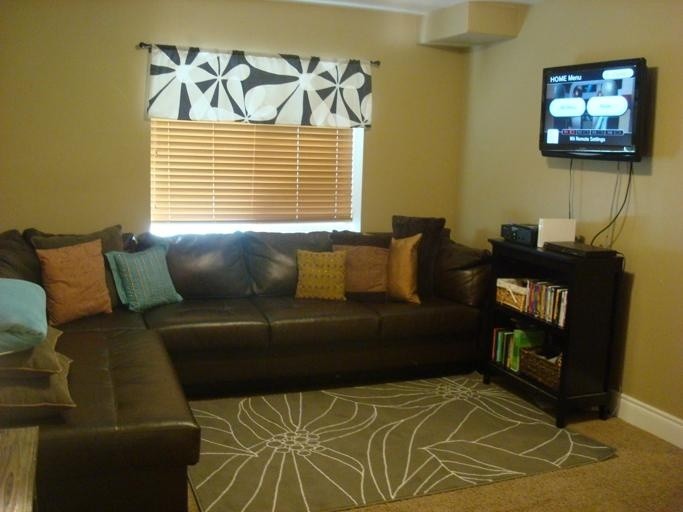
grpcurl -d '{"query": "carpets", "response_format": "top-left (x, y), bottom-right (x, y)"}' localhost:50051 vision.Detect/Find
top-left (182, 371), bottom-right (617, 512)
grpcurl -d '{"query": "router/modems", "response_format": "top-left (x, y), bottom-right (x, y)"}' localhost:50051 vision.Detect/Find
top-left (537, 218), bottom-right (576, 249)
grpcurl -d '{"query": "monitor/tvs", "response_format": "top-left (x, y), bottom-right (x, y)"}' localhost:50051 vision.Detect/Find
top-left (538, 57), bottom-right (647, 163)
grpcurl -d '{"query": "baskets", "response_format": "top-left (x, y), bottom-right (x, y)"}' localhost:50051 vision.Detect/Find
top-left (495, 278), bottom-right (538, 314)
top-left (519, 345), bottom-right (562, 391)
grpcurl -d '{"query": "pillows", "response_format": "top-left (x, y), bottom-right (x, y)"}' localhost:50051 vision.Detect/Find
top-left (331, 244), bottom-right (390, 295)
top-left (242, 229), bottom-right (332, 302)
top-left (1, 225), bottom-right (183, 418)
top-left (295, 249), bottom-right (347, 302)
top-left (137, 232), bottom-right (241, 302)
top-left (392, 216), bottom-right (445, 297)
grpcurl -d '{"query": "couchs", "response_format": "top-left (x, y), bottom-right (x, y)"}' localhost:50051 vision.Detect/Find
top-left (1, 228), bottom-right (493, 512)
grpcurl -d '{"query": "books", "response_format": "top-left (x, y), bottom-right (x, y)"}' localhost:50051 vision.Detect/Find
top-left (491, 326), bottom-right (544, 374)
top-left (524, 278), bottom-right (567, 330)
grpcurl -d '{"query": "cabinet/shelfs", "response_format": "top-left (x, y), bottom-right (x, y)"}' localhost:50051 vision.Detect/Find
top-left (484, 237), bottom-right (624, 428)
